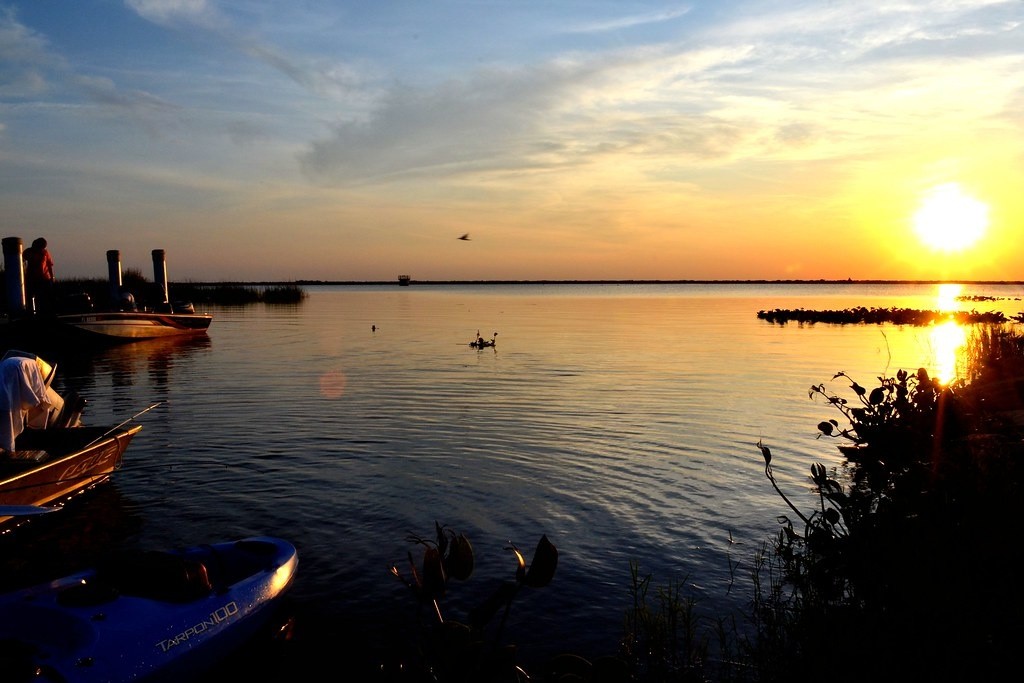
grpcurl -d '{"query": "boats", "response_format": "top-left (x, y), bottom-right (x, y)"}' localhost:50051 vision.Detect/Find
top-left (1, 537), bottom-right (297, 683)
top-left (68, 313), bottom-right (212, 338)
top-left (0, 425), bottom-right (144, 538)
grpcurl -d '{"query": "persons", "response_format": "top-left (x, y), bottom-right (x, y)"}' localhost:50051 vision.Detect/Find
top-left (22, 238), bottom-right (54, 314)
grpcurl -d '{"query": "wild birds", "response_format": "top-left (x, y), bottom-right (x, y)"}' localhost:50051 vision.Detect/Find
top-left (457, 233), bottom-right (470, 241)
